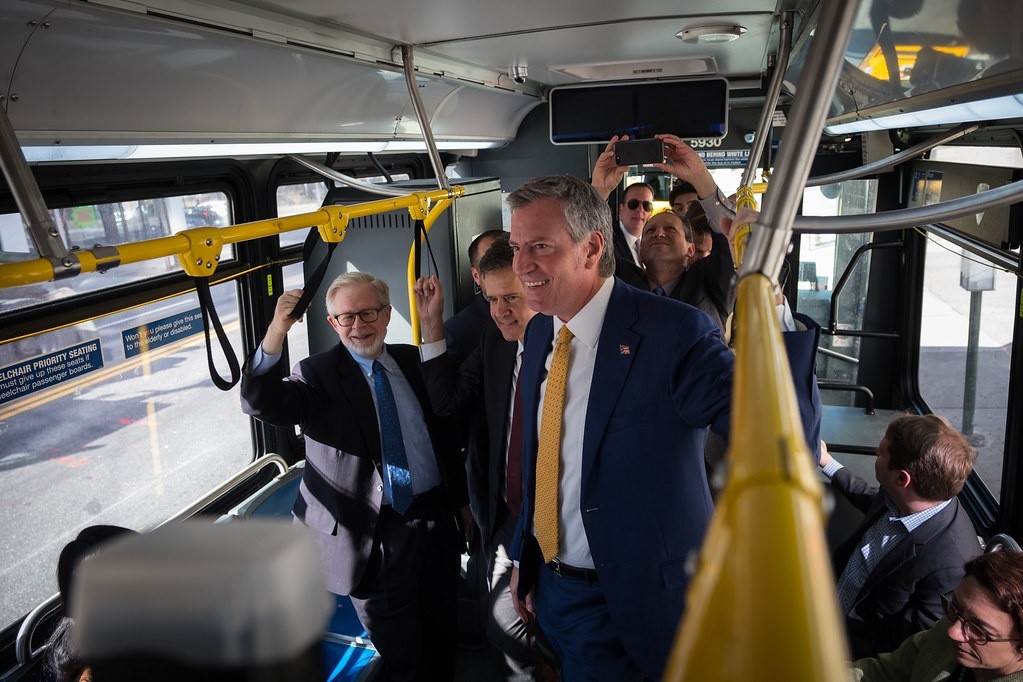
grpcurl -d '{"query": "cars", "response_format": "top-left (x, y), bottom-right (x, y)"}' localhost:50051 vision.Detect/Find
top-left (129, 202), bottom-right (219, 236)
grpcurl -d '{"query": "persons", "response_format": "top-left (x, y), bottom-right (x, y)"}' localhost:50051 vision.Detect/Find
top-left (817, 414), bottom-right (987, 661)
top-left (237, 132), bottom-right (831, 682)
top-left (845, 544), bottom-right (1023, 682)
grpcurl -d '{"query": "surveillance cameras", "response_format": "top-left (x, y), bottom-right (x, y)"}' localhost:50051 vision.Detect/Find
top-left (507, 67), bottom-right (528, 84)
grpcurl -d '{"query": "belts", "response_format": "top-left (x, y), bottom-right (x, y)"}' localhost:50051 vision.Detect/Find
top-left (536, 549), bottom-right (600, 579)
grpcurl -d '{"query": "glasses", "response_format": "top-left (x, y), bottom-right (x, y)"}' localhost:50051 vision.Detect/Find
top-left (331, 303), bottom-right (388, 327)
top-left (942, 589), bottom-right (1021, 646)
top-left (624, 198), bottom-right (652, 212)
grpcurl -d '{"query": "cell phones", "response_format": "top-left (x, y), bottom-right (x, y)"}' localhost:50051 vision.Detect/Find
top-left (614, 137), bottom-right (665, 166)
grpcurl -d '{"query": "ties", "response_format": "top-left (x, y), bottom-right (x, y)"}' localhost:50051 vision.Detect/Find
top-left (534, 326), bottom-right (573, 564)
top-left (635, 239), bottom-right (644, 270)
top-left (371, 360), bottom-right (414, 516)
